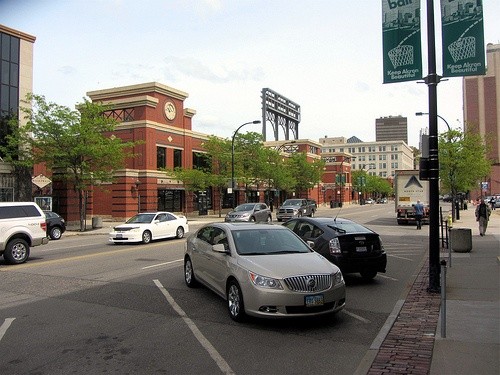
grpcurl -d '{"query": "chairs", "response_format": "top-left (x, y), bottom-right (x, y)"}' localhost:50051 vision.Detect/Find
top-left (299, 225), bottom-right (310, 235)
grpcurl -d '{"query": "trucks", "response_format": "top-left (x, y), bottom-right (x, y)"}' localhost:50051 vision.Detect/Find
top-left (393, 169), bottom-right (439, 224)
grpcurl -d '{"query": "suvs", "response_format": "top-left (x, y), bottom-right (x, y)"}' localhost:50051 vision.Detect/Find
top-left (0, 201), bottom-right (48, 264)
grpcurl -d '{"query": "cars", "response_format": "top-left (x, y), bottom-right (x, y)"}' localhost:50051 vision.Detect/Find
top-left (109, 211), bottom-right (188, 244)
top-left (162, 190), bottom-right (207, 200)
top-left (182, 222), bottom-right (347, 323)
top-left (439, 194), bottom-right (453, 203)
top-left (365, 198), bottom-right (388, 204)
top-left (44, 211), bottom-right (66, 240)
top-left (484, 196), bottom-right (500, 208)
top-left (283, 218), bottom-right (388, 281)
top-left (224, 202), bottom-right (273, 224)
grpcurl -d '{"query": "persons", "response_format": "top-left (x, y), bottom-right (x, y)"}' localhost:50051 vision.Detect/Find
top-left (463, 198), bottom-right (468, 210)
top-left (490, 197), bottom-right (496, 210)
top-left (475, 199), bottom-right (491, 237)
top-left (414, 200), bottom-right (424, 229)
top-left (270, 198), bottom-right (275, 212)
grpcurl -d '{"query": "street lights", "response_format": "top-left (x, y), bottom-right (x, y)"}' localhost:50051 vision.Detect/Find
top-left (267, 140), bottom-right (297, 204)
top-left (339, 156), bottom-right (357, 207)
top-left (230, 121), bottom-right (261, 208)
top-left (415, 113), bottom-right (457, 221)
top-left (359, 163), bottom-right (376, 206)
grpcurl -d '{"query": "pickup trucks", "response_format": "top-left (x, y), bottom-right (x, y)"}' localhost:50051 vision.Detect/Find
top-left (276, 199), bottom-right (316, 222)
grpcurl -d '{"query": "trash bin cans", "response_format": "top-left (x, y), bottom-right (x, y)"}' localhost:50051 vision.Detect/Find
top-left (91, 217), bottom-right (104, 229)
top-left (451, 227), bottom-right (473, 253)
